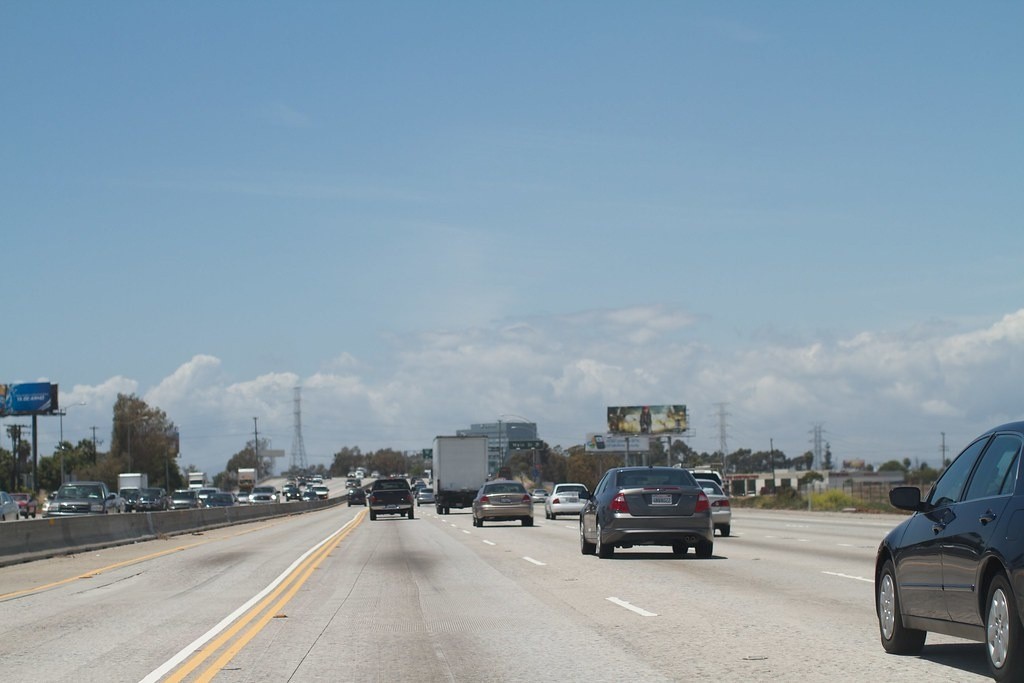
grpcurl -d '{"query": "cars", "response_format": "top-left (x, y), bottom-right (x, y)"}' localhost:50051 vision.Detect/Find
top-left (199, 487), bottom-right (220, 499)
top-left (346, 478), bottom-right (357, 488)
top-left (205, 493), bottom-right (240, 509)
top-left (108, 493), bottom-right (126, 513)
top-left (249, 485), bottom-right (285, 508)
top-left (532, 487), bottom-right (550, 502)
top-left (171, 490), bottom-right (200, 509)
top-left (356, 478), bottom-right (362, 486)
top-left (367, 464), bottom-right (409, 480)
top-left (695, 480), bottom-right (731, 536)
top-left (283, 485), bottom-right (295, 494)
top-left (578, 466), bottom-right (716, 559)
top-left (348, 471), bottom-right (355, 477)
top-left (418, 488), bottom-right (435, 505)
top-left (743, 490), bottom-right (754, 497)
top-left (545, 482), bottom-right (594, 520)
top-left (303, 491), bottom-right (318, 501)
top-left (287, 488), bottom-right (303, 502)
top-left (313, 485), bottom-right (341, 496)
top-left (348, 488), bottom-right (367, 508)
top-left (9, 493), bottom-right (38, 518)
top-left (474, 480), bottom-right (535, 528)
top-left (0, 491), bottom-right (20, 522)
top-left (285, 463), bottom-right (333, 484)
top-left (411, 471), bottom-right (426, 498)
top-left (237, 492), bottom-right (250, 503)
top-left (872, 420), bottom-right (1024, 683)
top-left (43, 494), bottom-right (53, 517)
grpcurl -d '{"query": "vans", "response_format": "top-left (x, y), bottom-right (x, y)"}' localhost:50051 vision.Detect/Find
top-left (684, 470), bottom-right (727, 496)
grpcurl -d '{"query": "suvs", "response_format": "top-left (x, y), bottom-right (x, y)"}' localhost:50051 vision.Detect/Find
top-left (136, 487), bottom-right (170, 510)
top-left (48, 481), bottom-right (109, 517)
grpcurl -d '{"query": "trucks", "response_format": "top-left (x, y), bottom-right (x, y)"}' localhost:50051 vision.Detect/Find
top-left (119, 472), bottom-right (148, 511)
top-left (237, 468), bottom-right (257, 492)
top-left (356, 467), bottom-right (367, 478)
top-left (431, 437), bottom-right (491, 514)
top-left (188, 472), bottom-right (207, 489)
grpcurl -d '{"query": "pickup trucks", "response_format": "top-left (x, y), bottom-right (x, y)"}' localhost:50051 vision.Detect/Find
top-left (368, 478), bottom-right (415, 520)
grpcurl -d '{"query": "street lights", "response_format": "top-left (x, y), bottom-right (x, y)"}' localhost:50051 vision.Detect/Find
top-left (60, 402), bottom-right (87, 486)
